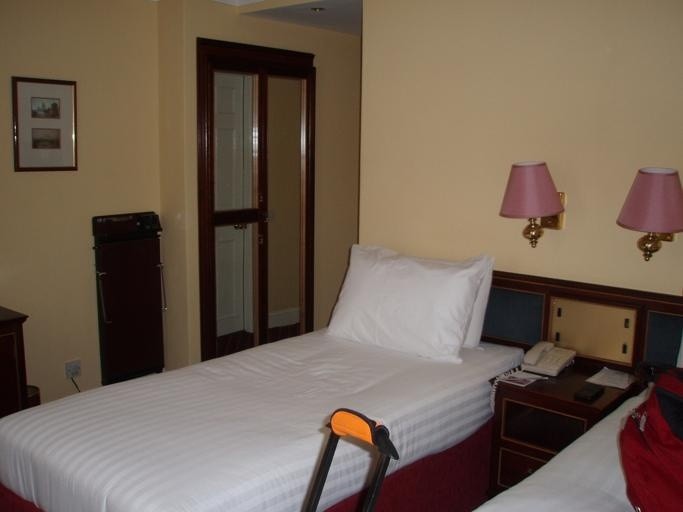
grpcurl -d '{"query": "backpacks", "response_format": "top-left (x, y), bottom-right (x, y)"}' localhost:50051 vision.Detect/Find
top-left (616, 366), bottom-right (683, 512)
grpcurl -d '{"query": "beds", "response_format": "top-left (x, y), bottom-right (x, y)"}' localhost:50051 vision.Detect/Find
top-left (0, 281), bottom-right (552, 512)
top-left (467, 382), bottom-right (682, 511)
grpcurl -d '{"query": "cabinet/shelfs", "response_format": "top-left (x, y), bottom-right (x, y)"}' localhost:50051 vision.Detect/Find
top-left (0, 306), bottom-right (30, 419)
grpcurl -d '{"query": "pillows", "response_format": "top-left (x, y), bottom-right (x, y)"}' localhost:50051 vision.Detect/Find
top-left (327, 243), bottom-right (493, 362)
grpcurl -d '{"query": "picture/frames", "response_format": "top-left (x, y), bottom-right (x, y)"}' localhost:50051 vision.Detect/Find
top-left (11, 75), bottom-right (79, 173)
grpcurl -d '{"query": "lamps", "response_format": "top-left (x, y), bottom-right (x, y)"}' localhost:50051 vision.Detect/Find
top-left (497, 160), bottom-right (564, 250)
top-left (616, 168), bottom-right (683, 261)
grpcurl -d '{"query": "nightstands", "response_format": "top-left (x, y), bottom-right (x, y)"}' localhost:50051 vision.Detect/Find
top-left (490, 361), bottom-right (645, 497)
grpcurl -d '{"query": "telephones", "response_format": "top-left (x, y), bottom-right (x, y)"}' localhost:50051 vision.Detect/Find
top-left (522, 341), bottom-right (577, 376)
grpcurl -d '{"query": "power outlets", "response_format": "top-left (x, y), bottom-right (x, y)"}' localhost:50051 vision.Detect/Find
top-left (65, 358), bottom-right (81, 380)
